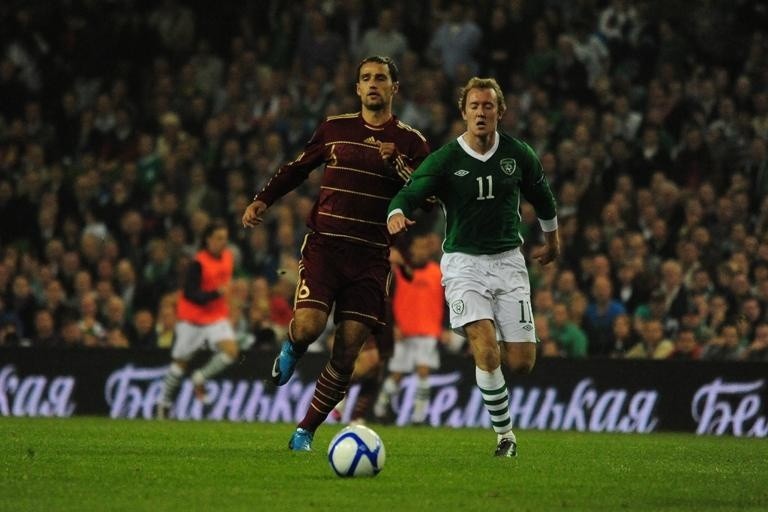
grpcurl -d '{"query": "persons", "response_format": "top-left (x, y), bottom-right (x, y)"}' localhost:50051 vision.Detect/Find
top-left (382, 73), bottom-right (560, 457)
top-left (155, 221), bottom-right (241, 426)
top-left (239, 53), bottom-right (433, 450)
top-left (366, 233), bottom-right (449, 430)
top-left (2, 0), bottom-right (766, 364)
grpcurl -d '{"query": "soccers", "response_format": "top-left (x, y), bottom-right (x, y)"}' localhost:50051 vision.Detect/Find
top-left (328, 424), bottom-right (386, 478)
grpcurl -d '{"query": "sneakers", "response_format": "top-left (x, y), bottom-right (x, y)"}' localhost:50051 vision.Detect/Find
top-left (288, 426), bottom-right (315, 453)
top-left (271, 335), bottom-right (302, 386)
top-left (492, 435), bottom-right (516, 460)
